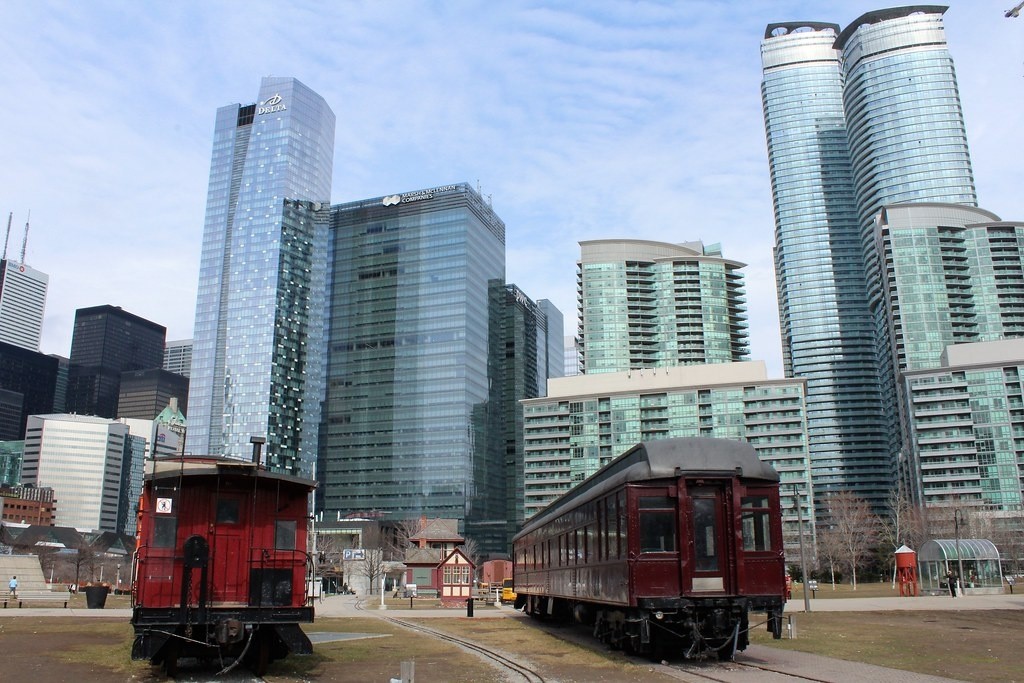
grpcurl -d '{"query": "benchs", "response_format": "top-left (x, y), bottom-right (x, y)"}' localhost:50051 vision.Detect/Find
top-left (0, 591), bottom-right (11, 608)
top-left (17, 591), bottom-right (71, 609)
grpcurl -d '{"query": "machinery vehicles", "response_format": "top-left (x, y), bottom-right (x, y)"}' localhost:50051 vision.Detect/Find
top-left (477, 577), bottom-right (517, 605)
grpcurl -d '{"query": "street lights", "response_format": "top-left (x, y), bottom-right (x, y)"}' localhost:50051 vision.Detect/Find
top-left (953, 509), bottom-right (969, 595)
top-left (792, 484), bottom-right (810, 612)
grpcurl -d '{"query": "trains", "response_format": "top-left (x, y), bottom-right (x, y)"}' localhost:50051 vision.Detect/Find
top-left (132, 456), bottom-right (315, 677)
top-left (512, 438), bottom-right (785, 659)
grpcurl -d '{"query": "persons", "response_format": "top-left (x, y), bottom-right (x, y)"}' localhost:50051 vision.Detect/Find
top-left (793, 580), bottom-right (798, 591)
top-left (70, 582), bottom-right (76, 594)
top-left (343, 583), bottom-right (348, 595)
top-left (947, 571), bottom-right (956, 597)
top-left (9, 576), bottom-right (17, 599)
